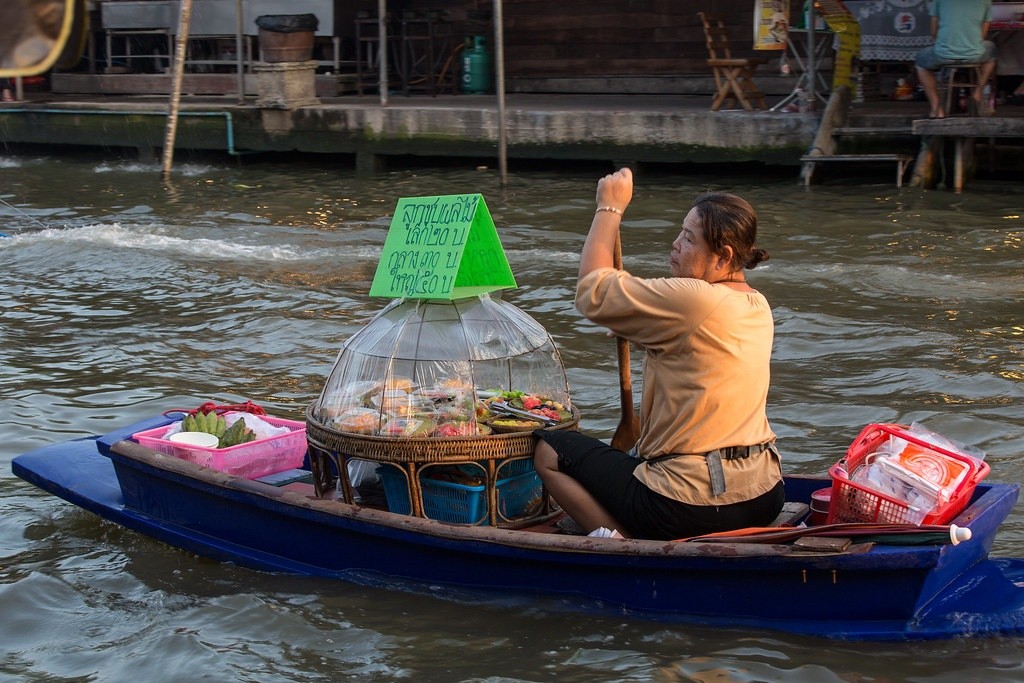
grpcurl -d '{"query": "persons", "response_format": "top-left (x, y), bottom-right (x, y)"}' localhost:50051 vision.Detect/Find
top-left (0, 78), bottom-right (15, 101)
top-left (533, 169), bottom-right (789, 540)
top-left (915, 0), bottom-right (997, 119)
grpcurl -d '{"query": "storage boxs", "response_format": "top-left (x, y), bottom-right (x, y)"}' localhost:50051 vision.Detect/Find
top-left (133, 411), bottom-right (308, 480)
top-left (372, 459), bottom-right (543, 526)
top-left (823, 422), bottom-right (990, 529)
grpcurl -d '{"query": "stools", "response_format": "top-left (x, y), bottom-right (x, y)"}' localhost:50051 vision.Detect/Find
top-left (943, 63), bottom-right (990, 116)
top-left (252, 62), bottom-right (319, 107)
top-left (769, 29), bottom-right (835, 112)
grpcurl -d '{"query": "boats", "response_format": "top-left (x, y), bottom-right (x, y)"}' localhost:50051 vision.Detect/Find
top-left (9, 404), bottom-right (1023, 646)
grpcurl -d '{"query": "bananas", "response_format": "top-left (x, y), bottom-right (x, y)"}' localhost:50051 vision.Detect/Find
top-left (182, 411), bottom-right (256, 449)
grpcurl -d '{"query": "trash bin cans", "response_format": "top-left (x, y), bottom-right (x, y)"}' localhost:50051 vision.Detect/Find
top-left (257, 13), bottom-right (318, 64)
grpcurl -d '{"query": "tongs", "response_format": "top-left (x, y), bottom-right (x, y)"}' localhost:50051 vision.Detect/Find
top-left (489, 400), bottom-right (558, 427)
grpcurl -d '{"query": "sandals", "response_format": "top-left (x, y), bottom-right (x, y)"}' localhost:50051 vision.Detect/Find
top-left (929, 102), bottom-right (944, 118)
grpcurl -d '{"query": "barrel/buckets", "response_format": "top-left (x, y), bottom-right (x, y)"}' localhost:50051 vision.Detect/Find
top-left (809, 487), bottom-right (835, 526)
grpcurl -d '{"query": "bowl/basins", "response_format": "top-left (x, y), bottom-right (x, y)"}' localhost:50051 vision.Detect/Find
top-left (170, 432), bottom-right (219, 461)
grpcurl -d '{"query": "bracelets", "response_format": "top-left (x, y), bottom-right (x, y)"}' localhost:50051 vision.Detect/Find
top-left (595, 204), bottom-right (624, 218)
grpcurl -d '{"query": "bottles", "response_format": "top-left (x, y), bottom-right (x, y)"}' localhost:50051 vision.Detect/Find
top-left (801, 0), bottom-right (809, 29)
top-left (958, 88), bottom-right (967, 113)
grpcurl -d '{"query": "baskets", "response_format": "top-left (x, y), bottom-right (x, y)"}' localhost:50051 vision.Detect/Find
top-left (132, 410), bottom-right (308, 480)
top-left (374, 457), bottom-right (543, 525)
top-left (825, 423), bottom-right (991, 527)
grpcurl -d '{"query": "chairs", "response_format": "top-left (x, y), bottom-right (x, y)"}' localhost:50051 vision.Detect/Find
top-left (700, 9), bottom-right (753, 110)
